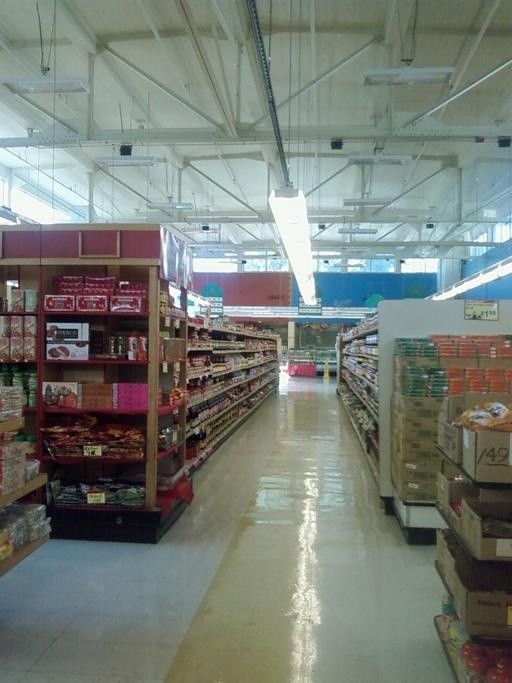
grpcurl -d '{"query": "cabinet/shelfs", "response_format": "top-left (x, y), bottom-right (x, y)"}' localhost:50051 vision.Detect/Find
top-left (286, 344), bottom-right (320, 381)
top-left (0, 223), bottom-right (282, 578)
top-left (335, 300), bottom-right (393, 518)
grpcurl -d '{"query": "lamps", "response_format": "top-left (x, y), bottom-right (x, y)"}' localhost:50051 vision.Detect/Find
top-left (335, 247), bottom-right (370, 254)
top-left (145, 202), bottom-right (193, 212)
top-left (181, 227), bottom-right (219, 235)
top-left (95, 157), bottom-right (154, 168)
top-left (337, 227), bottom-right (377, 235)
top-left (342, 197), bottom-right (393, 207)
top-left (358, 65), bottom-right (456, 87)
top-left (4, 78), bottom-right (90, 96)
top-left (346, 153), bottom-right (413, 167)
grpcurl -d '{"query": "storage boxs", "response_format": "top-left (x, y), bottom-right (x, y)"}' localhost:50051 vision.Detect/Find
top-left (394, 355), bottom-right (512, 638)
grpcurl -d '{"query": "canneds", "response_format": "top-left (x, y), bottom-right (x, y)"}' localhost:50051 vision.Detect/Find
top-left (1, 285), bottom-right (39, 461)
